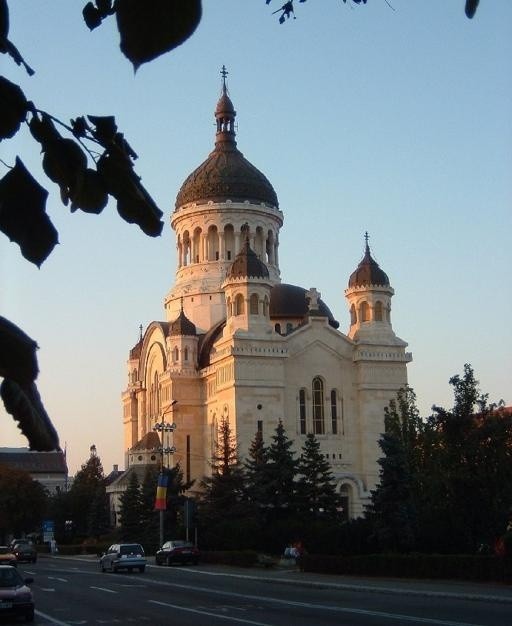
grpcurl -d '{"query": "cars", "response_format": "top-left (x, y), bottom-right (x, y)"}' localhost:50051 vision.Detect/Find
top-left (0, 546), bottom-right (18, 567)
top-left (99, 543), bottom-right (147, 573)
top-left (0, 564), bottom-right (35, 621)
top-left (155, 539), bottom-right (200, 567)
top-left (12, 543), bottom-right (37, 563)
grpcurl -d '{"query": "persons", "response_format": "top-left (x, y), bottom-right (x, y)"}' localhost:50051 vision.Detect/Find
top-left (50, 537), bottom-right (56, 556)
top-left (291, 539), bottom-right (305, 574)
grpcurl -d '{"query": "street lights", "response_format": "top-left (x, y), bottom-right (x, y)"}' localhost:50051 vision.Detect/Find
top-left (160, 398), bottom-right (179, 548)
